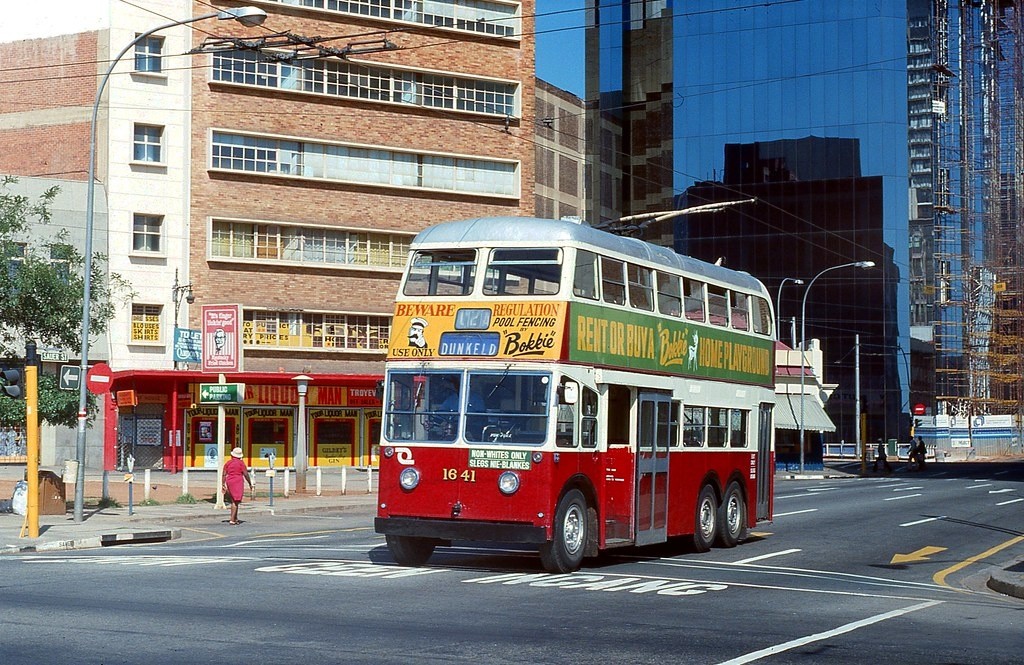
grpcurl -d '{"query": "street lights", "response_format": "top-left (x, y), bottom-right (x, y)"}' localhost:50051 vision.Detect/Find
top-left (800, 261), bottom-right (877, 478)
top-left (72, 6), bottom-right (266, 525)
top-left (778, 278), bottom-right (804, 339)
top-left (291, 375), bottom-right (314, 494)
top-left (172, 282), bottom-right (196, 472)
top-left (833, 343), bottom-right (860, 460)
top-left (183, 407), bottom-right (200, 498)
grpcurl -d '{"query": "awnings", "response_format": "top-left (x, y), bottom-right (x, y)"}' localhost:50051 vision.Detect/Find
top-left (683, 394), bottom-right (836, 432)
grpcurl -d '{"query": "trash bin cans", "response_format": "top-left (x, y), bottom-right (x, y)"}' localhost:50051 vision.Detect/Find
top-left (866, 448), bottom-right (874, 461)
top-left (24, 470), bottom-right (66, 516)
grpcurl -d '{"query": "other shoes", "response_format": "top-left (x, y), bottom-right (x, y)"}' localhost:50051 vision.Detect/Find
top-left (230, 519), bottom-right (239, 524)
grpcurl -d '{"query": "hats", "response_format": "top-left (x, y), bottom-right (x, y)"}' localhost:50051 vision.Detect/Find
top-left (231, 447), bottom-right (243, 458)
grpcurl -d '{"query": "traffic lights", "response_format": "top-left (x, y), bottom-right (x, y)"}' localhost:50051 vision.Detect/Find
top-left (915, 419), bottom-right (922, 428)
top-left (0, 367), bottom-right (25, 399)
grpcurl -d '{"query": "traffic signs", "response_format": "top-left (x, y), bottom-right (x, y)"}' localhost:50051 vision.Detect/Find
top-left (199, 381), bottom-right (240, 404)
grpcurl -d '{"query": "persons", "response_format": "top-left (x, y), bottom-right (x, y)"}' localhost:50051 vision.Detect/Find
top-left (0, 429), bottom-right (17, 456)
top-left (907, 436), bottom-right (926, 471)
top-left (435, 375), bottom-right (484, 420)
top-left (873, 439), bottom-right (892, 473)
top-left (222, 448), bottom-right (253, 525)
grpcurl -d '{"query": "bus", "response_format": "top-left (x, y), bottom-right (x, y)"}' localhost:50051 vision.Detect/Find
top-left (376, 214), bottom-right (777, 572)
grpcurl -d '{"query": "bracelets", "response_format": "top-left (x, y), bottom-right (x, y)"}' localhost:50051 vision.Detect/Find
top-left (250, 483), bottom-right (251, 485)
top-left (223, 485), bottom-right (225, 486)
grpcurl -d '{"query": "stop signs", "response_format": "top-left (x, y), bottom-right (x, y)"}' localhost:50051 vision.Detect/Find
top-left (85, 363), bottom-right (112, 395)
top-left (914, 403), bottom-right (924, 415)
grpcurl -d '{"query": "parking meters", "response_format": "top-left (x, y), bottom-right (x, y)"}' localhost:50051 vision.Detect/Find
top-left (267, 453), bottom-right (277, 504)
top-left (127, 453), bottom-right (136, 516)
